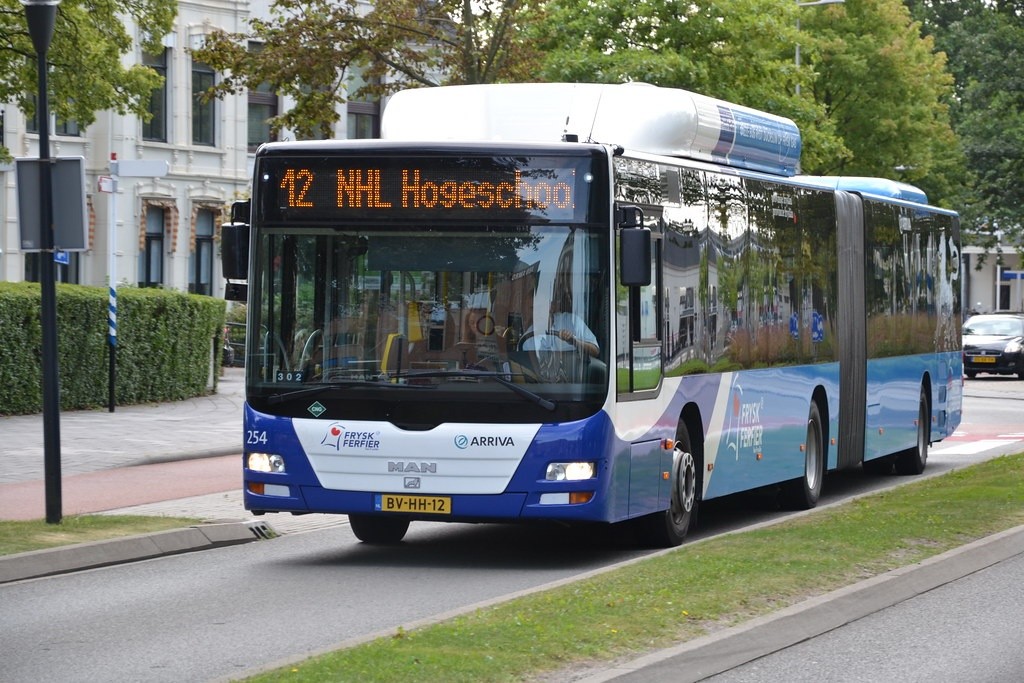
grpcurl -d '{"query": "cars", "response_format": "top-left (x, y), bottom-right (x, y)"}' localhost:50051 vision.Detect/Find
top-left (960, 314), bottom-right (1023, 377)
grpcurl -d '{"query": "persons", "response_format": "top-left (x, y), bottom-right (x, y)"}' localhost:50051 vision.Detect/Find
top-left (295, 329), bottom-right (313, 361)
top-left (517, 287), bottom-right (599, 356)
top-left (464, 304), bottom-right (507, 372)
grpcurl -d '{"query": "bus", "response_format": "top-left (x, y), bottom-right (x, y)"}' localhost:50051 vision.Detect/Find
top-left (220, 80), bottom-right (968, 542)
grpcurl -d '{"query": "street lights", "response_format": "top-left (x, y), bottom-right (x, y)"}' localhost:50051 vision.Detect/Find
top-left (20, 0), bottom-right (65, 522)
